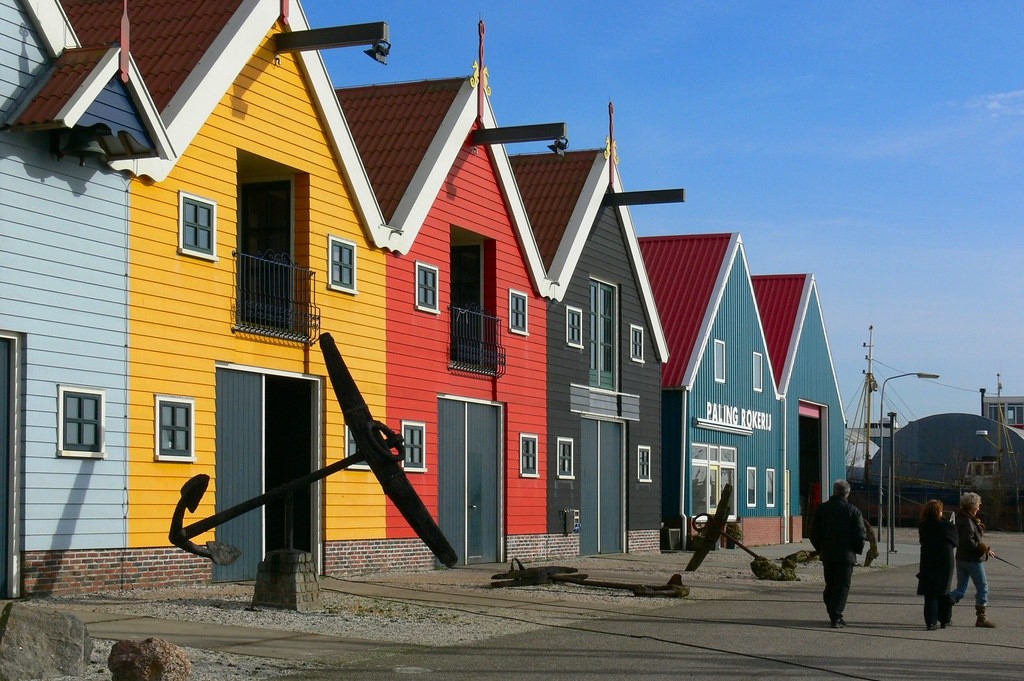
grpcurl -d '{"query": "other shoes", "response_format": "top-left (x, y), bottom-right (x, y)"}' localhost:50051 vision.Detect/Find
top-left (937, 616), bottom-right (954, 625)
top-left (927, 623), bottom-right (937, 630)
top-left (829, 613), bottom-right (846, 628)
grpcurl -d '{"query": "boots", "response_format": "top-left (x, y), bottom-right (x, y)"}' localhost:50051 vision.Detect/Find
top-left (975, 605), bottom-right (997, 628)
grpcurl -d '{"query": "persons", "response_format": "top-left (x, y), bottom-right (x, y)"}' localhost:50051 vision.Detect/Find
top-left (916, 499), bottom-right (958, 631)
top-left (809, 480), bottom-right (867, 628)
top-left (949, 492), bottom-right (998, 628)
